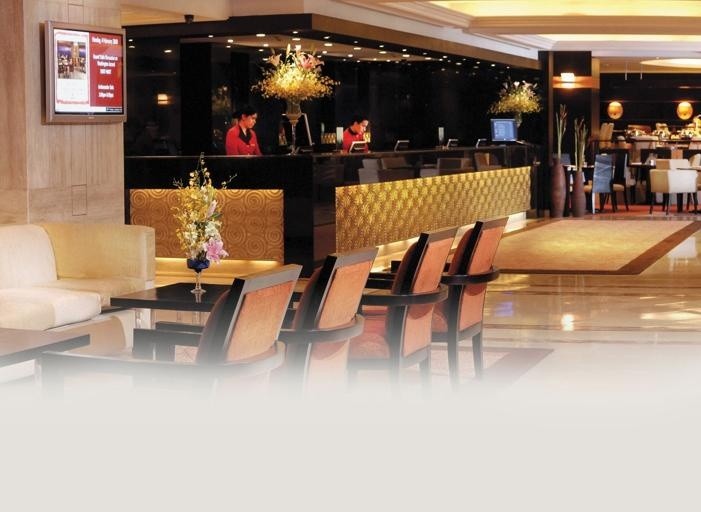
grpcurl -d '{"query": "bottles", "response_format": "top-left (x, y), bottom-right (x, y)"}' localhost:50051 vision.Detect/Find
top-left (321, 133), bottom-right (336, 143)
top-left (216, 85), bottom-right (228, 112)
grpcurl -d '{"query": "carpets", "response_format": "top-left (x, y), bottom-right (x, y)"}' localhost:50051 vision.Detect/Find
top-left (384, 211), bottom-right (701, 279)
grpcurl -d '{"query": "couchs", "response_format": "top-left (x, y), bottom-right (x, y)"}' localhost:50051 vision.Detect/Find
top-left (0, 217), bottom-right (164, 387)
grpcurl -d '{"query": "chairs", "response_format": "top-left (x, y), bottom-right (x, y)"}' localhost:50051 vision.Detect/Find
top-left (289, 220), bottom-right (462, 399)
top-left (352, 214), bottom-right (512, 397)
top-left (34, 263), bottom-right (304, 398)
top-left (357, 151), bottom-right (508, 187)
top-left (154, 245), bottom-right (382, 395)
top-left (570, 112), bottom-right (700, 218)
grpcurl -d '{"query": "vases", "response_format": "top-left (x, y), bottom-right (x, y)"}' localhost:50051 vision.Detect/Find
top-left (549, 161), bottom-right (568, 220)
top-left (507, 111), bottom-right (524, 129)
top-left (285, 97), bottom-right (306, 158)
top-left (185, 246), bottom-right (212, 293)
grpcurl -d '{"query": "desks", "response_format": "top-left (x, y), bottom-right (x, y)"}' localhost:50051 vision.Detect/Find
top-left (0, 326), bottom-right (91, 376)
top-left (108, 279), bottom-right (296, 358)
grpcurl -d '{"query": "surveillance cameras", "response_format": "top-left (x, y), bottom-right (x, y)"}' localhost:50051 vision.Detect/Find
top-left (184, 15), bottom-right (195, 24)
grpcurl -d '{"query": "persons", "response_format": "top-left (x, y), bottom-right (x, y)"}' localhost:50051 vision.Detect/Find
top-left (225, 106), bottom-right (262, 155)
top-left (343, 113), bottom-right (369, 153)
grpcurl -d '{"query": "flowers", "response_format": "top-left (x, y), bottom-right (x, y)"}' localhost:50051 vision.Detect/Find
top-left (486, 74), bottom-right (545, 116)
top-left (247, 40), bottom-right (343, 104)
top-left (165, 149), bottom-right (244, 268)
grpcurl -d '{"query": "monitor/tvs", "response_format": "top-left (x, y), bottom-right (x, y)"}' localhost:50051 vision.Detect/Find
top-left (476, 138), bottom-right (488, 147)
top-left (349, 141), bottom-right (366, 153)
top-left (394, 139), bottom-right (410, 151)
top-left (282, 111), bottom-right (313, 153)
top-left (490, 118), bottom-right (518, 143)
top-left (446, 139), bottom-right (459, 147)
